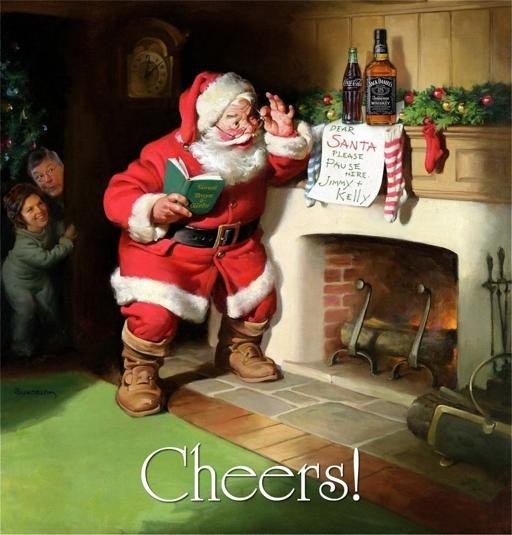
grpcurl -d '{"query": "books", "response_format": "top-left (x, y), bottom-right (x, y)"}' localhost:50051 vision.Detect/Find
top-left (158, 153), bottom-right (225, 218)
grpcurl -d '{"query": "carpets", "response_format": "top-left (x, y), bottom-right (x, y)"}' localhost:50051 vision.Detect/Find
top-left (2, 368), bottom-right (448, 535)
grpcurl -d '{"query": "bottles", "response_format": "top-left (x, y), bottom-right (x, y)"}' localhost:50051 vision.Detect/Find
top-left (360, 28), bottom-right (402, 125)
top-left (340, 45), bottom-right (367, 124)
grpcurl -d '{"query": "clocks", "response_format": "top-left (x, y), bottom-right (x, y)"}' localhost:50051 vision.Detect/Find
top-left (112, 16), bottom-right (185, 111)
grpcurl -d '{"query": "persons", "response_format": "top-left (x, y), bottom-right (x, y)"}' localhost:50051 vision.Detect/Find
top-left (21, 142), bottom-right (67, 200)
top-left (1, 184), bottom-right (79, 363)
top-left (100, 68), bottom-right (316, 418)
